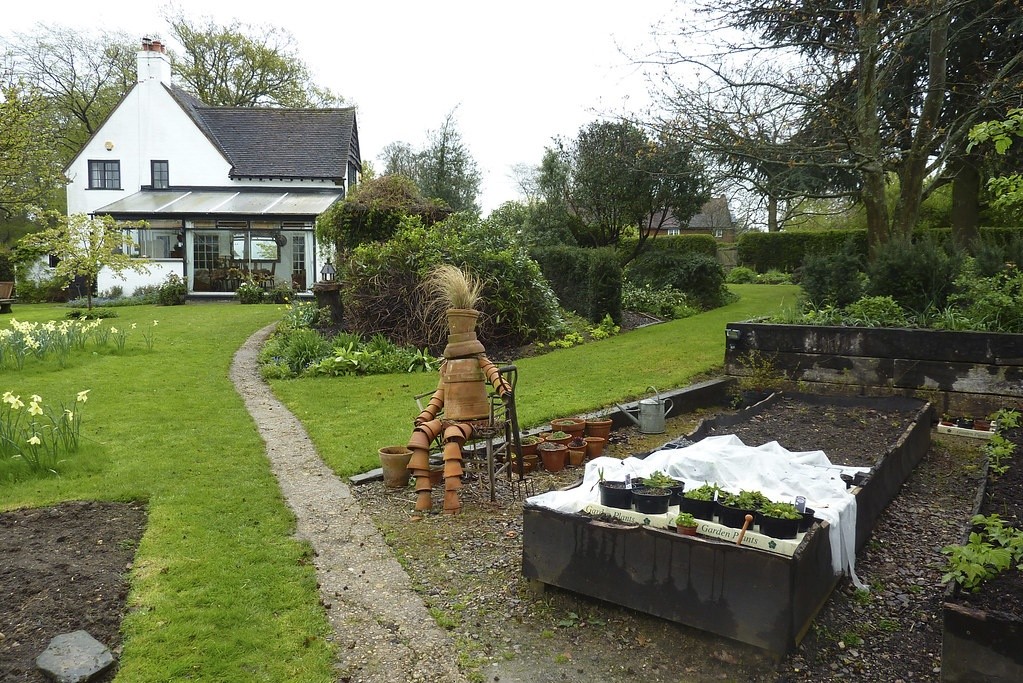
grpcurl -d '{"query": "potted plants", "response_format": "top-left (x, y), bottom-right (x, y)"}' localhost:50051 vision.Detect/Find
top-left (422, 261), bottom-right (491, 334)
top-left (942, 413), bottom-right (997, 431)
top-left (590, 469), bottom-right (815, 539)
top-left (504, 404), bottom-right (613, 474)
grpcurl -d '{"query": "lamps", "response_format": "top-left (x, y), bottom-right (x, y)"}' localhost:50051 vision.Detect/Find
top-left (272, 232), bottom-right (288, 246)
top-left (320, 257), bottom-right (336, 281)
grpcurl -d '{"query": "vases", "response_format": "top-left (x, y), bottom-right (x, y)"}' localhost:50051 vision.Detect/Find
top-left (378, 446), bottom-right (413, 487)
top-left (405, 333), bottom-right (512, 511)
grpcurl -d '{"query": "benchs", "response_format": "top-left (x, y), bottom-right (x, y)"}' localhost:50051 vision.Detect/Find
top-left (0, 282), bottom-right (15, 314)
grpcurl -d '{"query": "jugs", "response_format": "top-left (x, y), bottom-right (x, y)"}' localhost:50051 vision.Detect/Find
top-left (615, 386), bottom-right (674, 435)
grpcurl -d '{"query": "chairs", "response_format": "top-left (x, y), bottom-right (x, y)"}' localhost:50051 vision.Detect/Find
top-left (195, 268), bottom-right (275, 290)
top-left (413, 359), bottom-right (513, 502)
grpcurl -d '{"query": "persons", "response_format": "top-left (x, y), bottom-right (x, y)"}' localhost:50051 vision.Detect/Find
top-left (407, 265), bottom-right (513, 522)
